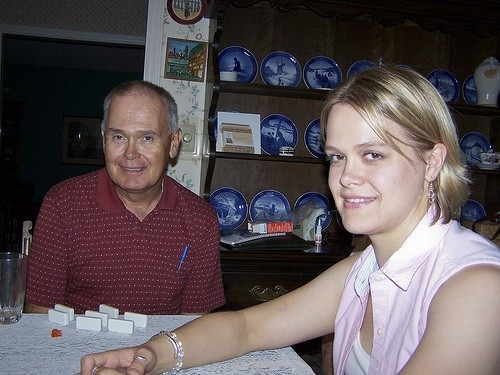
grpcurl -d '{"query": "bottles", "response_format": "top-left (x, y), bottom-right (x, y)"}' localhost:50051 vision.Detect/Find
top-left (474, 56), bottom-right (500, 107)
top-left (313, 218), bottom-right (323, 246)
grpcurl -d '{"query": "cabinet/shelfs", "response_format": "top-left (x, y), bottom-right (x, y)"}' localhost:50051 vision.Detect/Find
top-left (204, 44), bottom-right (500, 265)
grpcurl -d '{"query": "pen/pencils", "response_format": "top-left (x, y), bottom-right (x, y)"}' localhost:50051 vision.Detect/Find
top-left (178, 244), bottom-right (189, 271)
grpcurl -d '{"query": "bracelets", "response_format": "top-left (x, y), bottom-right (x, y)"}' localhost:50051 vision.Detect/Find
top-left (150, 330), bottom-right (184, 375)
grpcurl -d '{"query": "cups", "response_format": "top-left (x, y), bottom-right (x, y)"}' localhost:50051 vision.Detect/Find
top-left (0, 252), bottom-right (27, 324)
top-left (278, 146), bottom-right (295, 157)
top-left (220, 71), bottom-right (238, 82)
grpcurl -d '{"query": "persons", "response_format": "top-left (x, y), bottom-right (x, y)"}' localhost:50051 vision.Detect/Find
top-left (75, 64), bottom-right (500, 375)
top-left (17, 79), bottom-right (227, 315)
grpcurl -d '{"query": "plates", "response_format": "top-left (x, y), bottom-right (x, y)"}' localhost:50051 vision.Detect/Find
top-left (426, 68), bottom-right (460, 106)
top-left (260, 52), bottom-right (303, 87)
top-left (458, 132), bottom-right (500, 170)
top-left (301, 56), bottom-right (343, 89)
top-left (461, 199), bottom-right (486, 222)
top-left (346, 60), bottom-right (378, 81)
top-left (248, 189), bottom-right (292, 223)
top-left (293, 192), bottom-right (333, 234)
top-left (217, 46), bottom-right (258, 83)
top-left (304, 117), bottom-right (326, 158)
top-left (213, 110), bottom-right (241, 139)
top-left (396, 64), bottom-right (414, 71)
top-left (207, 187), bottom-right (248, 231)
top-left (259, 114), bottom-right (298, 155)
top-left (462, 74), bottom-right (478, 105)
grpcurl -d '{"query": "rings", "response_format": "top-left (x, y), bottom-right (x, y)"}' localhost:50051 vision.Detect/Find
top-left (135, 355), bottom-right (149, 367)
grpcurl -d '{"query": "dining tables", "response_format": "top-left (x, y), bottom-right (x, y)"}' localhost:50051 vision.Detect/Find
top-left (0, 313), bottom-right (316, 375)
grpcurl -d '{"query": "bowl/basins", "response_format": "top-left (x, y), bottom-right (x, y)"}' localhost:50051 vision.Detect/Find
top-left (479, 152), bottom-right (500, 165)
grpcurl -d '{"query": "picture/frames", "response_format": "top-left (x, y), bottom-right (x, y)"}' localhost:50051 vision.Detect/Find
top-left (2, 102), bottom-right (21, 166)
top-left (167, 0), bottom-right (207, 25)
top-left (163, 37), bottom-right (207, 82)
top-left (63, 115), bottom-right (106, 164)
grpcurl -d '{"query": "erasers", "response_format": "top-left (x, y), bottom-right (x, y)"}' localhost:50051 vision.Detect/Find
top-left (85, 310), bottom-right (109, 327)
top-left (48, 308), bottom-right (68, 325)
top-left (55, 304), bottom-right (74, 321)
top-left (108, 318), bottom-right (134, 334)
top-left (124, 312), bottom-right (149, 328)
top-left (99, 304), bottom-right (118, 320)
top-left (76, 316), bottom-right (102, 331)
